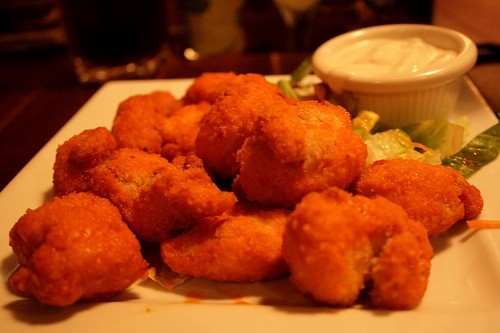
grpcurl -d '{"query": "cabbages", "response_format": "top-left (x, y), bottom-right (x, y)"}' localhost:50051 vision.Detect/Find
top-left (274, 60), bottom-right (443, 166)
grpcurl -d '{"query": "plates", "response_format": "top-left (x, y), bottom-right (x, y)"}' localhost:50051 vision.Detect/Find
top-left (0, 71), bottom-right (500, 333)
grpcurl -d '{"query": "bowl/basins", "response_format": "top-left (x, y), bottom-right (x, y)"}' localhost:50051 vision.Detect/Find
top-left (310, 23), bottom-right (477, 132)
